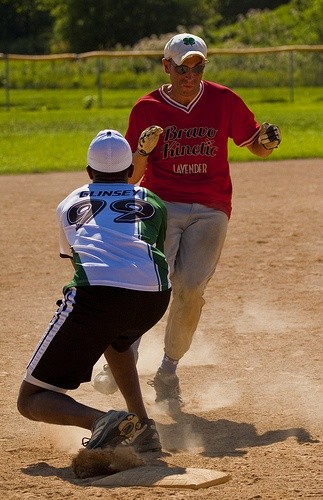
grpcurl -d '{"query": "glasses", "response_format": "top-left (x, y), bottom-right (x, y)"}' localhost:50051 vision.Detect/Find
top-left (171, 61), bottom-right (205, 74)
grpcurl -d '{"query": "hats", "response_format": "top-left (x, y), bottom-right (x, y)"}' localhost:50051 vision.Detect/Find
top-left (164, 34), bottom-right (207, 65)
top-left (87, 130), bottom-right (132, 172)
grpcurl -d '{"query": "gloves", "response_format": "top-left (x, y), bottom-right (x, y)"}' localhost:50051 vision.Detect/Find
top-left (258, 122), bottom-right (281, 150)
top-left (137, 125), bottom-right (163, 155)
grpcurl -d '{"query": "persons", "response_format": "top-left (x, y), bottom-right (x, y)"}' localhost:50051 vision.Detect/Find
top-left (17, 125), bottom-right (164, 453)
top-left (93, 32), bottom-right (281, 416)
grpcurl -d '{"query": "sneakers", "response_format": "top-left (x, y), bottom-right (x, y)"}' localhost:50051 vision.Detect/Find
top-left (76, 409), bottom-right (147, 478)
top-left (147, 367), bottom-right (182, 416)
top-left (92, 364), bottom-right (118, 394)
top-left (123, 419), bottom-right (162, 452)
